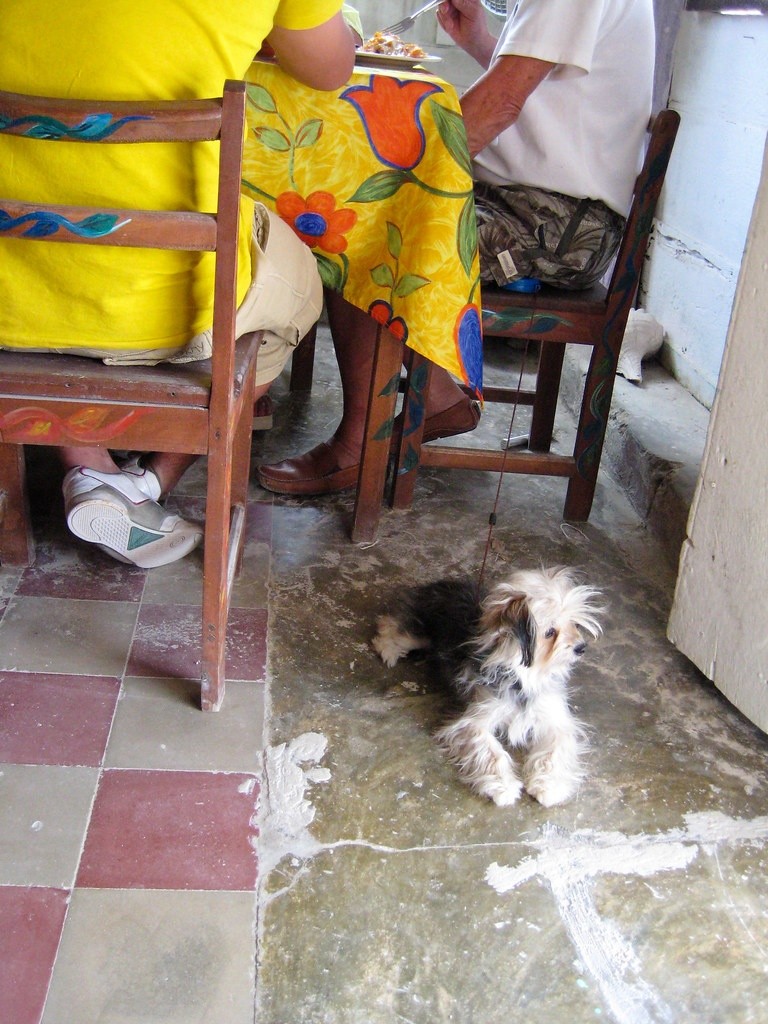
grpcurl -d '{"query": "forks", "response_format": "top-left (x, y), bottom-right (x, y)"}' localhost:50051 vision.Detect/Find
top-left (382, 0), bottom-right (447, 36)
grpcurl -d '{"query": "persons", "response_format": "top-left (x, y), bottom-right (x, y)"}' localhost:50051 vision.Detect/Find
top-left (255, 0), bottom-right (656, 496)
top-left (0, 0), bottom-right (365, 567)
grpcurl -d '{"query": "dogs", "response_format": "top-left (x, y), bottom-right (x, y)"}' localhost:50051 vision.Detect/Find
top-left (369, 562), bottom-right (607, 809)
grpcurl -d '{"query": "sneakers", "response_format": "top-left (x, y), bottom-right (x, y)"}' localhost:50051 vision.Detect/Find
top-left (62, 464), bottom-right (203, 569)
top-left (96, 455), bottom-right (170, 564)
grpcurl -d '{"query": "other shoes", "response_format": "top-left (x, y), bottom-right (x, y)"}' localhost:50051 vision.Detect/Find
top-left (253, 394), bottom-right (273, 430)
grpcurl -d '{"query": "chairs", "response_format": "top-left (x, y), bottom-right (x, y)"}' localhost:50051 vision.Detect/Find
top-left (393, 109), bottom-right (680, 522)
top-left (0, 80), bottom-right (266, 714)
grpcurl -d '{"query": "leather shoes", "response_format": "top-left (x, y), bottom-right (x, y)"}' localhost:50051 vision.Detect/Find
top-left (388, 394), bottom-right (481, 454)
top-left (256, 443), bottom-right (390, 495)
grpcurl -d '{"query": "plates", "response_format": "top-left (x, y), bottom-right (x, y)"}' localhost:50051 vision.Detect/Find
top-left (355, 49), bottom-right (442, 69)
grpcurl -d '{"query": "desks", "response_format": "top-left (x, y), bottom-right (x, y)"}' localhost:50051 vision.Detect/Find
top-left (239, 59), bottom-right (485, 542)
